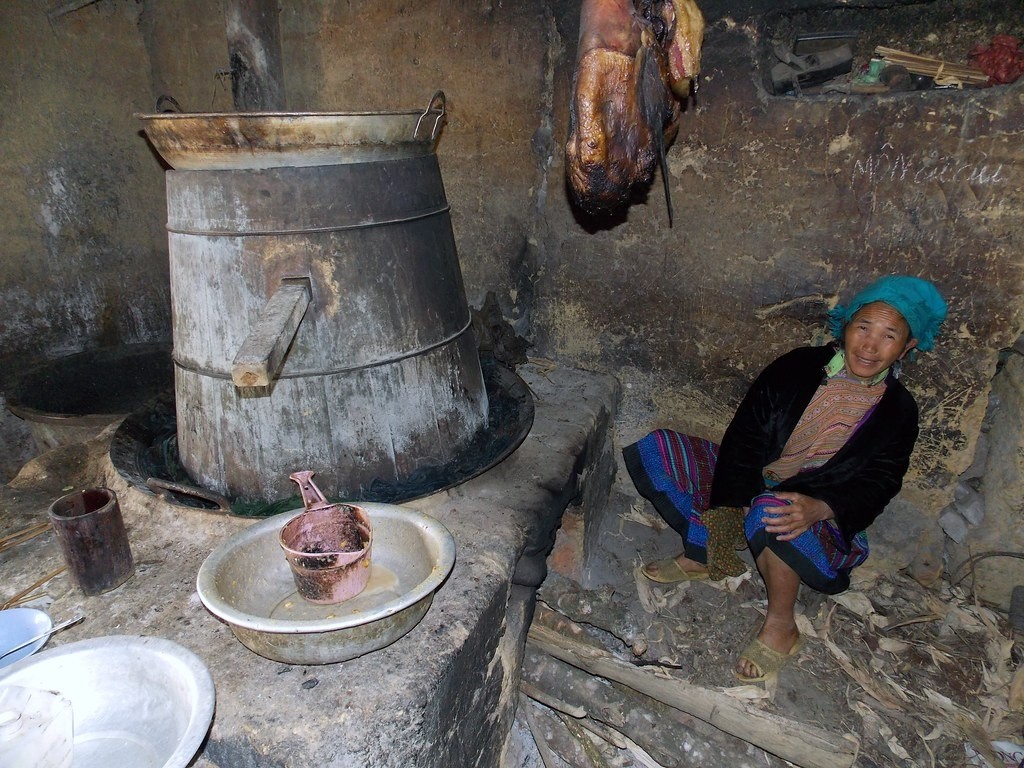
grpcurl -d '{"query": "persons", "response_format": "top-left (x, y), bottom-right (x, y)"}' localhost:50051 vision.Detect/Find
top-left (621, 277), bottom-right (947, 681)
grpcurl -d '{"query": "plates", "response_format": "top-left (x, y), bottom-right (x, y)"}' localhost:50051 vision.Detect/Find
top-left (0, 609), bottom-right (52, 668)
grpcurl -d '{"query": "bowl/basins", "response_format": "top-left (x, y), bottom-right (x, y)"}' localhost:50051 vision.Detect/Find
top-left (0, 636), bottom-right (215, 768)
top-left (196, 501), bottom-right (456, 665)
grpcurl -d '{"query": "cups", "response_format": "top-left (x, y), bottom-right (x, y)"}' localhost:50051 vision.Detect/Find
top-left (48, 487), bottom-right (135, 596)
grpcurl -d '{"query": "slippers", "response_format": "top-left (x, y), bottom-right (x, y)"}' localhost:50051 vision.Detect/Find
top-left (641, 556), bottom-right (710, 582)
top-left (735, 636), bottom-right (802, 683)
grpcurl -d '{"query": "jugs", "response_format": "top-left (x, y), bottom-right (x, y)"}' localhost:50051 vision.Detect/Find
top-left (279, 469), bottom-right (372, 604)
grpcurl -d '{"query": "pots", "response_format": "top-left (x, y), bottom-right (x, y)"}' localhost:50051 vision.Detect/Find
top-left (131, 92), bottom-right (450, 168)
